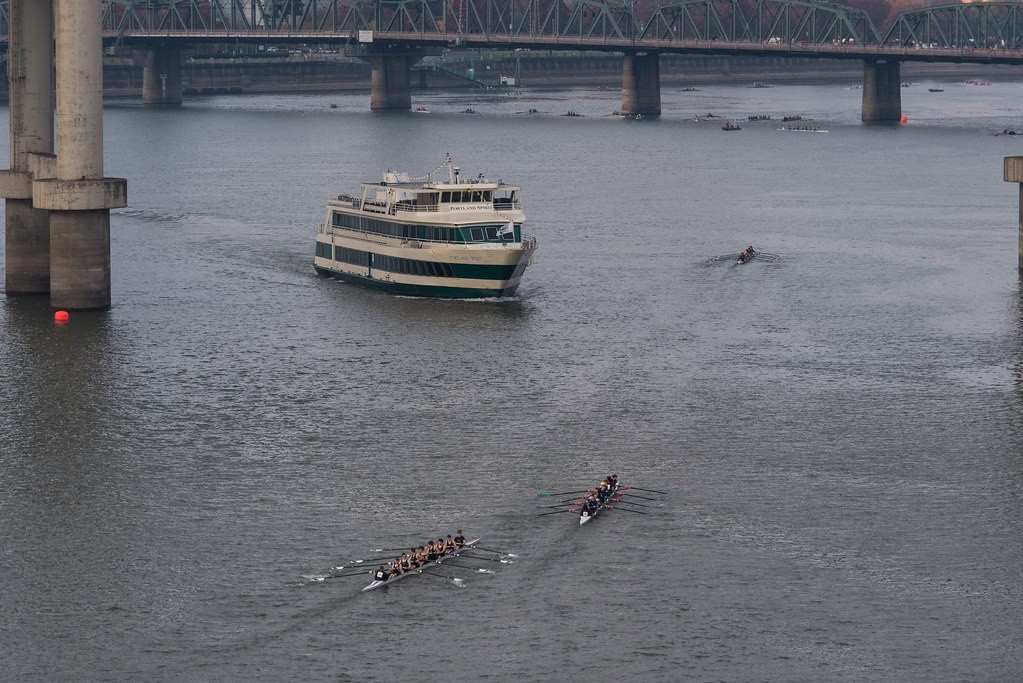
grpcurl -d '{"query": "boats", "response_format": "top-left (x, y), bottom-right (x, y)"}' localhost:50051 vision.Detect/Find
top-left (312, 149), bottom-right (538, 299)
top-left (988, 132), bottom-right (1023, 137)
top-left (721, 126), bottom-right (740, 130)
top-left (749, 117), bottom-right (772, 120)
top-left (516, 111), bottom-right (553, 114)
top-left (781, 118), bottom-right (814, 122)
top-left (456, 111), bottom-right (479, 114)
top-left (560, 114), bottom-right (584, 116)
top-left (578, 478), bottom-right (619, 527)
top-left (928, 88), bottom-right (944, 92)
top-left (600, 114), bottom-right (625, 117)
top-left (360, 536), bottom-right (484, 593)
top-left (777, 126), bottom-right (828, 133)
top-left (411, 109), bottom-right (431, 114)
top-left (736, 250), bottom-right (755, 264)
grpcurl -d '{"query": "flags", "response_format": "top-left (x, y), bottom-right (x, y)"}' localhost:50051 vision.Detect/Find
top-left (497, 222), bottom-right (513, 237)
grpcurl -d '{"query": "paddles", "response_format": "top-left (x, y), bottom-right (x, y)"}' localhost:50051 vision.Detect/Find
top-left (318, 541), bottom-right (518, 582)
top-left (533, 484), bottom-right (667, 515)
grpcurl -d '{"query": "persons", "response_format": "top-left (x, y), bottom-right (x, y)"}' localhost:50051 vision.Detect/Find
top-left (707, 113), bottom-right (712, 116)
top-left (752, 80), bottom-right (771, 88)
top-left (374, 530), bottom-right (465, 581)
top-left (784, 115), bottom-right (802, 122)
top-left (465, 107), bottom-right (474, 113)
top-left (748, 115), bottom-right (770, 120)
top-left (613, 111), bottom-right (644, 118)
top-left (416, 106), bottom-right (425, 111)
top-left (683, 87), bottom-right (696, 91)
top-left (738, 245), bottom-right (755, 262)
top-left (566, 110), bottom-right (580, 116)
top-left (789, 125), bottom-right (821, 131)
top-left (568, 474), bottom-right (630, 517)
top-left (726, 124), bottom-right (740, 128)
top-left (965, 78), bottom-right (992, 87)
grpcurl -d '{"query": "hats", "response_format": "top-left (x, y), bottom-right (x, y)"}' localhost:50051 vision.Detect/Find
top-left (457, 529), bottom-right (462, 533)
